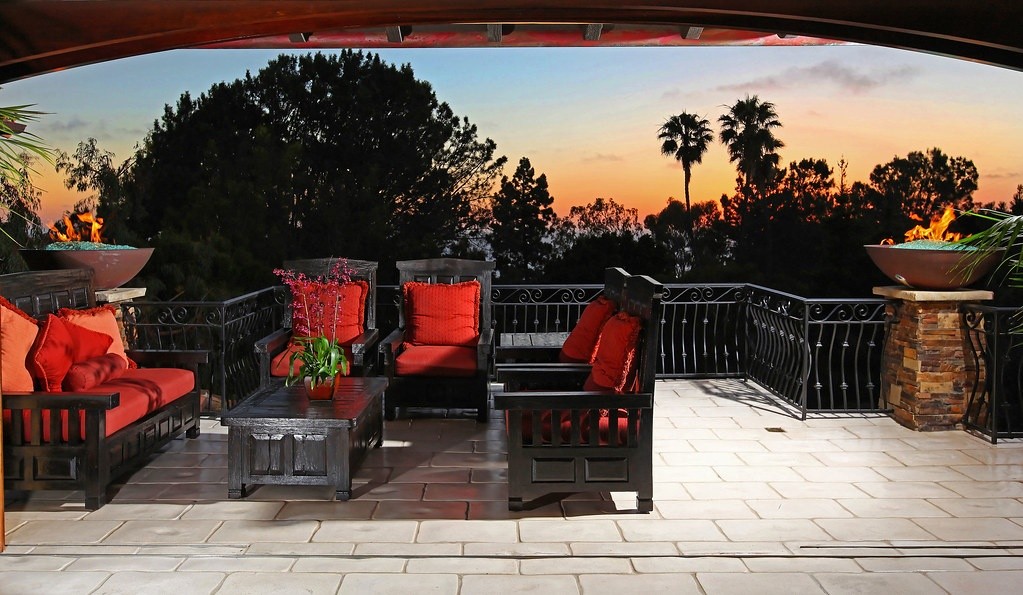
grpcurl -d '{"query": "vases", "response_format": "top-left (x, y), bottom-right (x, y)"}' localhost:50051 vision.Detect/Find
top-left (302, 375), bottom-right (338, 401)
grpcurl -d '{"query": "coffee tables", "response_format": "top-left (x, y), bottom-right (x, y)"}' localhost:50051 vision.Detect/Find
top-left (219, 377), bottom-right (390, 500)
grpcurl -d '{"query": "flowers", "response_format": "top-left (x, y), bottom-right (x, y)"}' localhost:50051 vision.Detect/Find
top-left (272, 260), bottom-right (359, 391)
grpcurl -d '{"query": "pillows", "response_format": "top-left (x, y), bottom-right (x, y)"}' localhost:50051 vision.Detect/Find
top-left (57, 304), bottom-right (137, 371)
top-left (60, 317), bottom-right (113, 363)
top-left (290, 281), bottom-right (368, 346)
top-left (0, 295), bottom-right (42, 392)
top-left (560, 296), bottom-right (615, 365)
top-left (68, 352), bottom-right (127, 392)
top-left (32, 313), bottom-right (76, 393)
top-left (403, 279), bottom-right (479, 346)
top-left (583, 312), bottom-right (639, 417)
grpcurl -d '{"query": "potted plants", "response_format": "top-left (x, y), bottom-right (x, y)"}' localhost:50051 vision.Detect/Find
top-left (938, 203), bottom-right (1023, 433)
top-left (864, 240), bottom-right (1008, 289)
top-left (17, 241), bottom-right (156, 291)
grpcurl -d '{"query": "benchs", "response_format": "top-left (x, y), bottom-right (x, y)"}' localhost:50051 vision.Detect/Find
top-left (0, 268), bottom-right (210, 510)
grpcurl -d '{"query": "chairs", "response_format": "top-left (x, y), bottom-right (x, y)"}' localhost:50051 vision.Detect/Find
top-left (493, 266), bottom-right (631, 443)
top-left (486, 275), bottom-right (671, 514)
top-left (253, 257), bottom-right (379, 385)
top-left (377, 258), bottom-right (494, 423)
